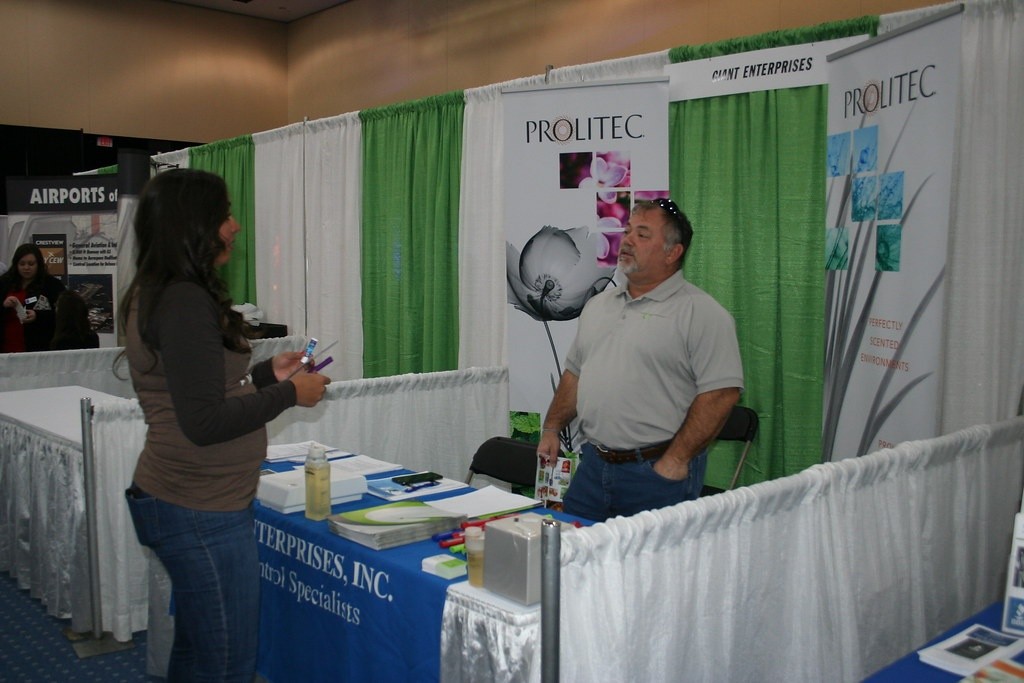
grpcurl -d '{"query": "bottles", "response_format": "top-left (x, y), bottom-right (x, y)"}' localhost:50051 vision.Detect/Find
top-left (459, 527), bottom-right (485, 588)
top-left (305, 442), bottom-right (330, 521)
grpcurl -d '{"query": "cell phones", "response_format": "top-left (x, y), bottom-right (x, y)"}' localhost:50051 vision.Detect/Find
top-left (393, 472), bottom-right (444, 486)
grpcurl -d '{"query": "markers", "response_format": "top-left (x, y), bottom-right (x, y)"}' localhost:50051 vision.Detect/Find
top-left (432, 513), bottom-right (521, 556)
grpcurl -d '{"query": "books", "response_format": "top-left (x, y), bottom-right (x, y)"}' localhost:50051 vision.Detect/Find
top-left (916, 496), bottom-right (1024, 683)
top-left (478, 502), bottom-right (544, 521)
top-left (326, 498), bottom-right (468, 551)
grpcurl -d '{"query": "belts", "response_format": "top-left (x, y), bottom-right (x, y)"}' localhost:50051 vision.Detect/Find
top-left (592, 443), bottom-right (670, 465)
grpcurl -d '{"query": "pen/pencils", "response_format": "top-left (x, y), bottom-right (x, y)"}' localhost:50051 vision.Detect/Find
top-left (403, 481), bottom-right (441, 493)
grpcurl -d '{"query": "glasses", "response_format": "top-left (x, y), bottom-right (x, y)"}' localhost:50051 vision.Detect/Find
top-left (651, 198), bottom-right (682, 219)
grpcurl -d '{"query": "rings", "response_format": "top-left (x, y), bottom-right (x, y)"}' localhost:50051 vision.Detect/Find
top-left (29, 315), bottom-right (31, 317)
top-left (321, 386), bottom-right (325, 393)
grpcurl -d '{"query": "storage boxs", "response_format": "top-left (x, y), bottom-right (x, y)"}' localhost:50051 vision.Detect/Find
top-left (254, 468), bottom-right (369, 514)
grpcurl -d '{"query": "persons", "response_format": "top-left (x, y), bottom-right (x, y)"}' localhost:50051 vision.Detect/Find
top-left (111, 167), bottom-right (332, 683)
top-left (0, 243), bottom-right (99, 353)
top-left (536, 197), bottom-right (744, 519)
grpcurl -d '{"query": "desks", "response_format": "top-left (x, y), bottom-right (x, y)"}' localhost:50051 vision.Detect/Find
top-left (0, 385), bottom-right (130, 632)
top-left (861, 598), bottom-right (1023, 683)
top-left (168, 459), bottom-right (601, 683)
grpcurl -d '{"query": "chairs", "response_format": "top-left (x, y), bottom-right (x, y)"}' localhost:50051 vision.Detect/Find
top-left (699, 405), bottom-right (759, 497)
top-left (465, 436), bottom-right (566, 508)
